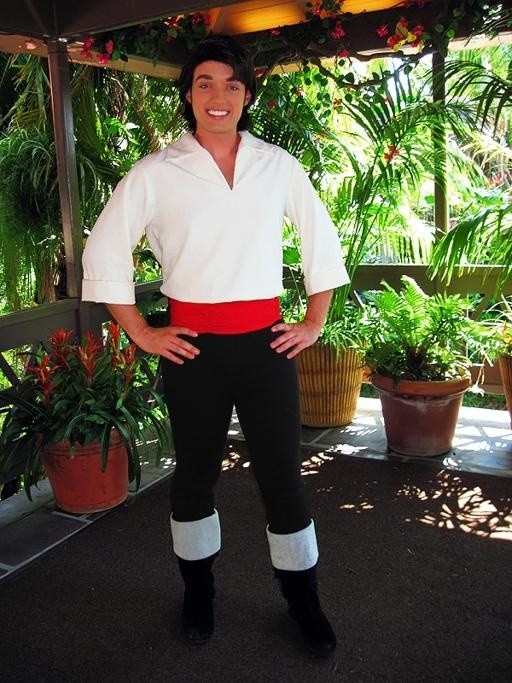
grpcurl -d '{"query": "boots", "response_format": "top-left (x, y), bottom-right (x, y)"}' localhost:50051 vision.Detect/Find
top-left (266, 519), bottom-right (336, 655)
top-left (170, 509), bottom-right (221, 644)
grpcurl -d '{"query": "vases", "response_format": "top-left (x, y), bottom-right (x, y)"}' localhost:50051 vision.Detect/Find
top-left (41, 423), bottom-right (130, 515)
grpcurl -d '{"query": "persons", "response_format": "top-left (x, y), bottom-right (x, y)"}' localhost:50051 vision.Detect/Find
top-left (80, 42), bottom-right (352, 658)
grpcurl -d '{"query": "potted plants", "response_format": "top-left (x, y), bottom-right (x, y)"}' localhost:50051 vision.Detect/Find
top-left (357, 275), bottom-right (481, 457)
top-left (475, 294), bottom-right (512, 435)
top-left (280, 159), bottom-right (399, 428)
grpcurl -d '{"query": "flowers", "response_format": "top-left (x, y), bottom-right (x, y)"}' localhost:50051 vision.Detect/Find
top-left (0, 323), bottom-right (176, 501)
